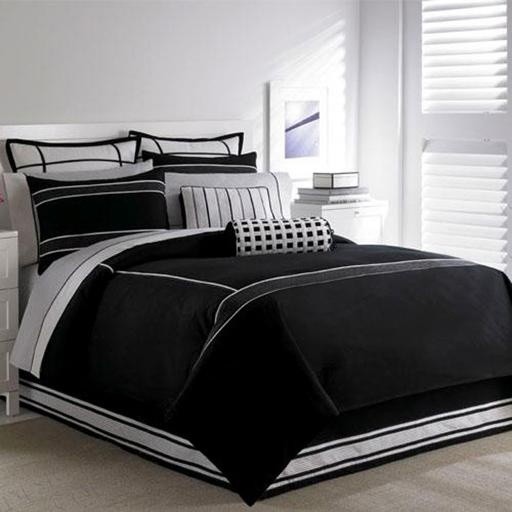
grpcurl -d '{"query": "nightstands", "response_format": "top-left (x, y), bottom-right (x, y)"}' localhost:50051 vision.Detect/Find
top-left (289, 200), bottom-right (389, 246)
top-left (0, 230), bottom-right (20, 417)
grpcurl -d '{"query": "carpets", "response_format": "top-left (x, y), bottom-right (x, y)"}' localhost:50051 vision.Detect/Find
top-left (1, 415), bottom-right (512, 511)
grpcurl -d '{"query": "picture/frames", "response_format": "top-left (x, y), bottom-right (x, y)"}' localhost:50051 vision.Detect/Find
top-left (269, 81), bottom-right (326, 172)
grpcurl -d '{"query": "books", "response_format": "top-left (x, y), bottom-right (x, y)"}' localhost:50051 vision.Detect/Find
top-left (292, 186), bottom-right (370, 205)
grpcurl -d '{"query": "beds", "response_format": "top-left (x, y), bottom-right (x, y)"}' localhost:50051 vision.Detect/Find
top-left (0, 120), bottom-right (512, 507)
